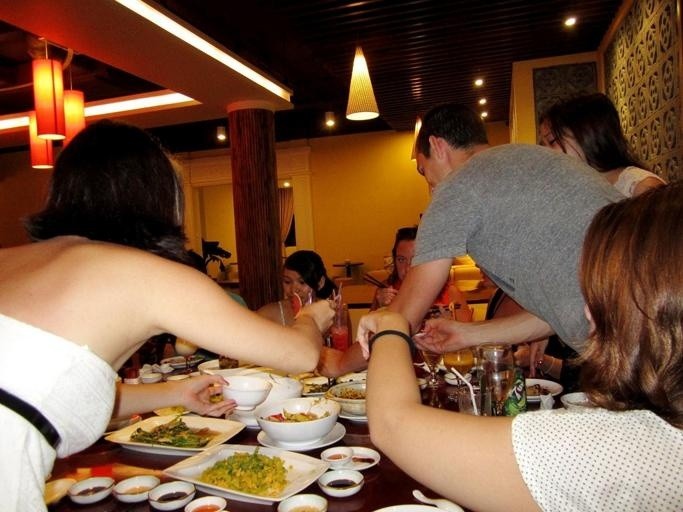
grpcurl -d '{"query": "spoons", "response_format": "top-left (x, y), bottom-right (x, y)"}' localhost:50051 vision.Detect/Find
top-left (412, 488), bottom-right (467, 512)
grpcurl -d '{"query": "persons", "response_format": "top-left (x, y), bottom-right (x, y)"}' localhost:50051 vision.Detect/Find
top-left (355, 183), bottom-right (682, 511)
top-left (251, 251), bottom-right (350, 367)
top-left (409, 90), bottom-right (665, 354)
top-left (367, 225), bottom-right (473, 324)
top-left (473, 263), bottom-right (522, 320)
top-left (513, 337), bottom-right (584, 391)
top-left (0, 119), bottom-right (337, 512)
top-left (314, 94), bottom-right (632, 379)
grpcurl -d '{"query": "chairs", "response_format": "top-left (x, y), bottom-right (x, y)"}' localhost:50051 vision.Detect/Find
top-left (45, 257), bottom-right (564, 511)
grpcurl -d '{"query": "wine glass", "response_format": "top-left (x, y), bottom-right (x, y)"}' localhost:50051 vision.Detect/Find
top-left (419, 340), bottom-right (512, 417)
top-left (173, 335), bottom-right (197, 373)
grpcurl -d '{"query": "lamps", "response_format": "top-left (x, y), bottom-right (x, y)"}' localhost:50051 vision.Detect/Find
top-left (21, 32), bottom-right (85, 171)
top-left (341, 30), bottom-right (382, 124)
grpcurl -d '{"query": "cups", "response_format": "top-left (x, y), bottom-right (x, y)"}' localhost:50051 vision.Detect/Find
top-left (329, 303), bottom-right (350, 353)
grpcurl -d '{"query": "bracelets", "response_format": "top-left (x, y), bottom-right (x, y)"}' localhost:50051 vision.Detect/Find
top-left (369, 329), bottom-right (414, 351)
top-left (544, 357), bottom-right (555, 375)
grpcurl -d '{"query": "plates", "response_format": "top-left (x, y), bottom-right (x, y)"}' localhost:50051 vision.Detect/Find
top-left (327, 382), bottom-right (372, 414)
top-left (322, 447), bottom-right (354, 466)
top-left (145, 481), bottom-right (195, 510)
top-left (331, 406), bottom-right (369, 423)
top-left (162, 443), bottom-right (330, 505)
top-left (115, 477), bottom-right (159, 504)
top-left (255, 422), bottom-right (347, 454)
top-left (319, 446), bottom-right (381, 474)
top-left (182, 495), bottom-right (227, 510)
top-left (109, 415), bottom-right (244, 455)
top-left (275, 495), bottom-right (328, 512)
top-left (515, 377), bottom-right (563, 403)
top-left (317, 469), bottom-right (365, 498)
top-left (558, 390), bottom-right (607, 409)
top-left (68, 475), bottom-right (112, 505)
top-left (107, 352), bottom-right (210, 383)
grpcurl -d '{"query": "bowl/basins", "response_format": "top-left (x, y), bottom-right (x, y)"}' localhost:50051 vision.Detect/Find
top-left (219, 375), bottom-right (273, 411)
top-left (232, 380), bottom-right (302, 425)
top-left (256, 396), bottom-right (342, 448)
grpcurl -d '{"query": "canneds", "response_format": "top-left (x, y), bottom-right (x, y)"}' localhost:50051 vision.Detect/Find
top-left (501, 365), bottom-right (528, 417)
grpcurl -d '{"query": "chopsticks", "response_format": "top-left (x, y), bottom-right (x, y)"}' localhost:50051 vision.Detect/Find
top-left (360, 273), bottom-right (387, 289)
